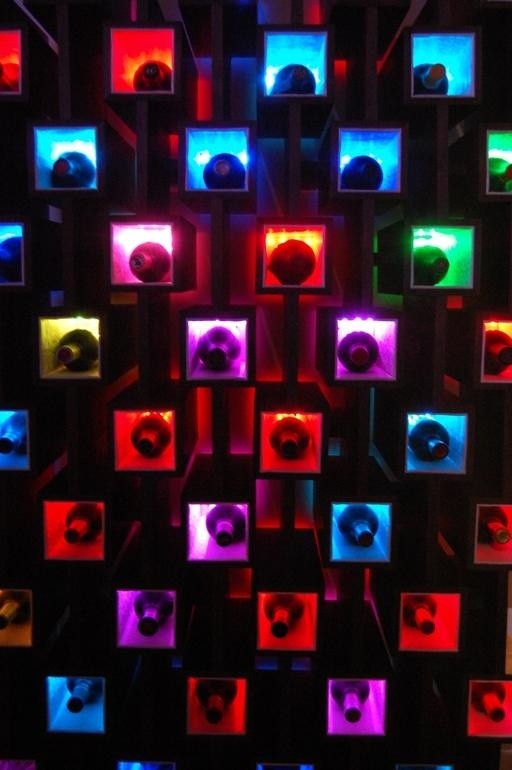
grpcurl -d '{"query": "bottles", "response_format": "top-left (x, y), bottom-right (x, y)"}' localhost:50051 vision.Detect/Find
top-left (1, 24), bottom-right (512, 728)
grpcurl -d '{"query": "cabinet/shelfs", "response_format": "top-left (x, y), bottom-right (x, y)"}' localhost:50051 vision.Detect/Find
top-left (0, 1), bottom-right (511, 770)
